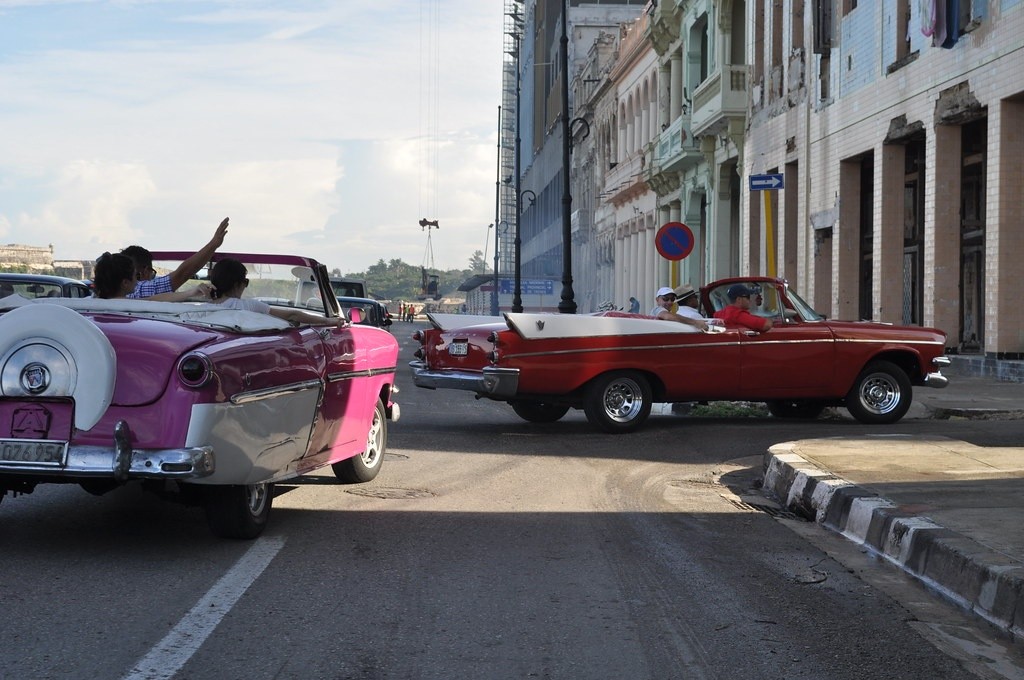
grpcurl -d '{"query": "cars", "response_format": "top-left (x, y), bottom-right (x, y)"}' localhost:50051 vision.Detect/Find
top-left (249, 265), bottom-right (394, 334)
top-left (406, 275), bottom-right (953, 437)
top-left (0, 271), bottom-right (95, 310)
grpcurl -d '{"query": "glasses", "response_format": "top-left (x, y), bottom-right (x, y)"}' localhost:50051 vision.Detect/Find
top-left (143, 266), bottom-right (157, 279)
top-left (661, 297), bottom-right (676, 302)
top-left (240, 278), bottom-right (250, 288)
top-left (742, 295), bottom-right (750, 300)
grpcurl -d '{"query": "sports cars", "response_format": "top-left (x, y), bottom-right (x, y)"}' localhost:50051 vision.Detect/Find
top-left (0, 250), bottom-right (401, 542)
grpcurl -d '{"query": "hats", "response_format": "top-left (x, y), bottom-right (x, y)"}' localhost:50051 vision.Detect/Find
top-left (726, 285), bottom-right (758, 299)
top-left (674, 282), bottom-right (699, 302)
top-left (656, 287), bottom-right (677, 297)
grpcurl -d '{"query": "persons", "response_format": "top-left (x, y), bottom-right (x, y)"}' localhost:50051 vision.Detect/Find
top-left (649, 283), bottom-right (726, 333)
top-left (713, 284), bottom-right (773, 331)
top-left (461, 304), bottom-right (466, 315)
top-left (629, 297), bottom-right (640, 313)
top-left (81, 217), bottom-right (347, 327)
top-left (398, 303), bottom-right (414, 323)
